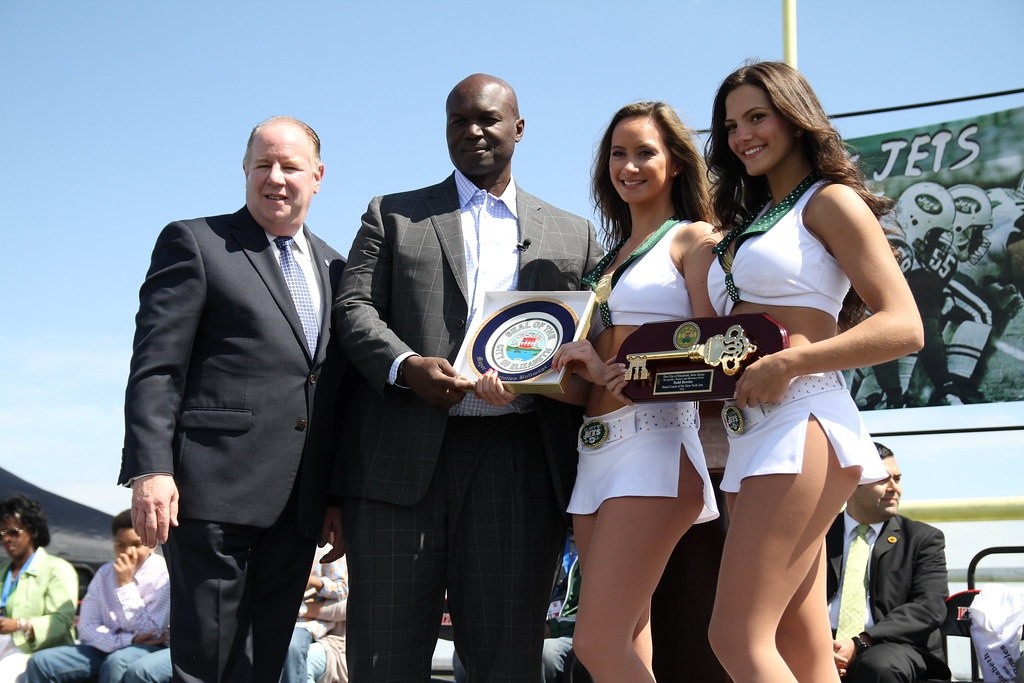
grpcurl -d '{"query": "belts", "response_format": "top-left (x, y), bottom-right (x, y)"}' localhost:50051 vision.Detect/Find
top-left (723, 371), bottom-right (843, 435)
top-left (578, 408), bottom-right (696, 450)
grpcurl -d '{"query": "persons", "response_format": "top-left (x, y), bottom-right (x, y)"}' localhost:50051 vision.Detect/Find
top-left (839, 180), bottom-right (1024, 414)
top-left (332, 75), bottom-right (605, 683)
top-left (701, 63), bottom-right (924, 683)
top-left (118, 115), bottom-right (347, 683)
top-left (0, 442), bottom-right (952, 683)
top-left (473, 103), bottom-right (724, 683)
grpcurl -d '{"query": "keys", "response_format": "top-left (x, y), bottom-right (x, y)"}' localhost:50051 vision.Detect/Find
top-left (624, 324), bottom-right (758, 382)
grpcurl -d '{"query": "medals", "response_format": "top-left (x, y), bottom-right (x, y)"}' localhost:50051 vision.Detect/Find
top-left (468, 298), bottom-right (580, 382)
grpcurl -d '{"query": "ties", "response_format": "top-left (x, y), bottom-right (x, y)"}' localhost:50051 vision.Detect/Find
top-left (835, 524), bottom-right (872, 645)
top-left (274, 235), bottom-right (320, 361)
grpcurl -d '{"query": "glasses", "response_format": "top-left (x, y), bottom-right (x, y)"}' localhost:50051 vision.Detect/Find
top-left (0, 528), bottom-right (23, 539)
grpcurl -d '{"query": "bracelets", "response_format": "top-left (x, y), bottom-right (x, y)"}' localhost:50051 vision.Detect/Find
top-left (17, 617), bottom-right (32, 636)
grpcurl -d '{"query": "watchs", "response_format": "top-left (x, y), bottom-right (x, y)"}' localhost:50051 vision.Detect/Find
top-left (851, 636), bottom-right (868, 655)
